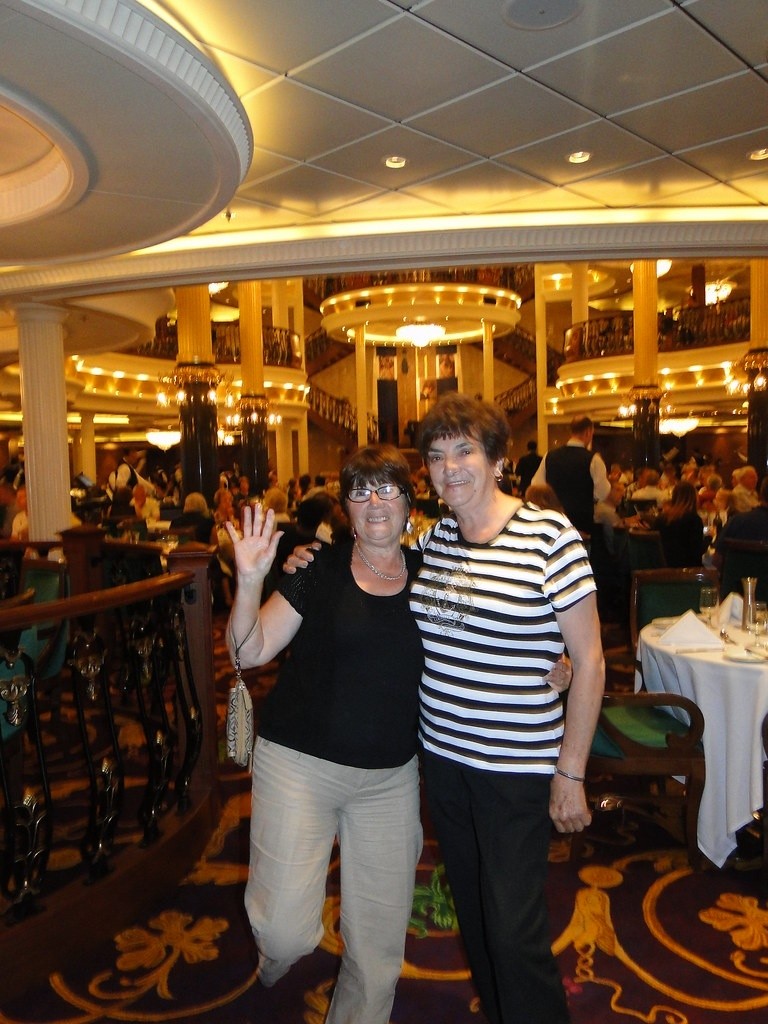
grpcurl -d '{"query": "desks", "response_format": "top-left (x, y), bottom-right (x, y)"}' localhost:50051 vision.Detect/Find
top-left (47, 491), bottom-right (712, 567)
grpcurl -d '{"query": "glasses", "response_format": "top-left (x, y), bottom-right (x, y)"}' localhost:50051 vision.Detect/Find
top-left (347, 485), bottom-right (406, 503)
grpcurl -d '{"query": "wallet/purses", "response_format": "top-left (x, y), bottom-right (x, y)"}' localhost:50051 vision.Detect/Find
top-left (224, 603), bottom-right (256, 775)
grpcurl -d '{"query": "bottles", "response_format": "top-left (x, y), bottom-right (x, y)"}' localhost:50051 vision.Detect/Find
top-left (742, 577), bottom-right (757, 631)
top-left (712, 510), bottom-right (722, 533)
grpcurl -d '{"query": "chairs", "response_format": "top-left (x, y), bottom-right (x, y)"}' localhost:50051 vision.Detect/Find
top-left (629, 568), bottom-right (721, 690)
top-left (716, 537), bottom-right (768, 610)
top-left (565, 687), bottom-right (705, 886)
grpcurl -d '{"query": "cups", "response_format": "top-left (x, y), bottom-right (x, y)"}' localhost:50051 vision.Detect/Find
top-left (754, 602), bottom-right (768, 632)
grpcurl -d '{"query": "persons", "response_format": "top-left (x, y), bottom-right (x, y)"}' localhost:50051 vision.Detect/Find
top-left (282, 394), bottom-right (605, 1024)
top-left (1, 356), bottom-right (768, 614)
top-left (223, 444), bottom-right (573, 1024)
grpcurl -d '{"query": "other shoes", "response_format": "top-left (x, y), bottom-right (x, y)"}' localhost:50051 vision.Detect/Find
top-left (255, 921), bottom-right (325, 988)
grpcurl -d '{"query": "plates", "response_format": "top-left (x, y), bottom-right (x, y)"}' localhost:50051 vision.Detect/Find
top-left (652, 617), bottom-right (679, 630)
top-left (724, 646), bottom-right (768, 663)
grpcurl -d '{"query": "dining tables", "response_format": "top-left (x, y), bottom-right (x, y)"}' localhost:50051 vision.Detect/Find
top-left (639, 614), bottom-right (768, 852)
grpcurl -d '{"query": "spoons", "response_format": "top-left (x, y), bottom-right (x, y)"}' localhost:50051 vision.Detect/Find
top-left (720, 630), bottom-right (737, 644)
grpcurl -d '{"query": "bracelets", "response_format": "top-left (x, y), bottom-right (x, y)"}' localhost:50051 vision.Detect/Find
top-left (555, 763), bottom-right (587, 785)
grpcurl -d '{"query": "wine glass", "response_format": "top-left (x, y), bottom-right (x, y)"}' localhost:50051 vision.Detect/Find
top-left (699, 588), bottom-right (720, 630)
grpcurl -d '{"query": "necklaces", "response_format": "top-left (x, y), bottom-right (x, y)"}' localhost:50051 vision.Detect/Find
top-left (354, 537), bottom-right (408, 581)
